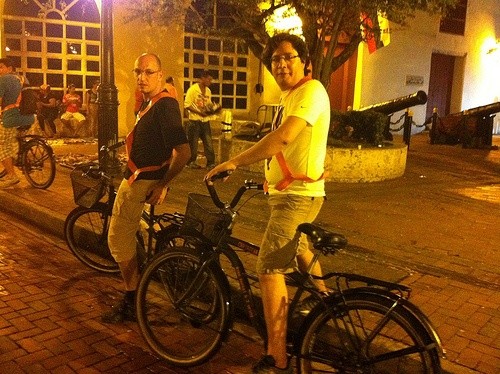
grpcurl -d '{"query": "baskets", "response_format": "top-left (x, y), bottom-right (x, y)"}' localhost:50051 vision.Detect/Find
top-left (178, 192), bottom-right (240, 245)
top-left (70, 162), bottom-right (106, 209)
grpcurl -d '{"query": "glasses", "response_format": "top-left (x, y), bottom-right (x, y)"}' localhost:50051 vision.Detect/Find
top-left (132, 69), bottom-right (160, 75)
top-left (268, 53), bottom-right (299, 61)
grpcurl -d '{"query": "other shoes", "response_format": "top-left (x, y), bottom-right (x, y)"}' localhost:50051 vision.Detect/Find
top-left (206, 165), bottom-right (215, 171)
top-left (0, 176), bottom-right (21, 189)
top-left (185, 161), bottom-right (201, 169)
top-left (101, 299), bottom-right (146, 323)
top-left (289, 289), bottom-right (333, 310)
top-left (224, 354), bottom-right (293, 374)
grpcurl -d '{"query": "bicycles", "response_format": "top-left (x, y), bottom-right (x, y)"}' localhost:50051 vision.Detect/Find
top-left (0, 124), bottom-right (57, 189)
top-left (63, 136), bottom-right (223, 323)
top-left (132, 168), bottom-right (449, 374)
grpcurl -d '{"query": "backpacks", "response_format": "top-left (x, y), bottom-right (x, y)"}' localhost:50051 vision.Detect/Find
top-left (20, 83), bottom-right (36, 115)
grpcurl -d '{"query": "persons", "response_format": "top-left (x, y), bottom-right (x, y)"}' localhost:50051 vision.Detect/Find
top-left (36, 83), bottom-right (59, 139)
top-left (60, 84), bottom-right (86, 137)
top-left (101, 52), bottom-right (192, 323)
top-left (183, 71), bottom-right (216, 170)
top-left (86, 80), bottom-right (100, 138)
top-left (203, 32), bottom-right (331, 374)
top-left (0, 58), bottom-right (35, 188)
